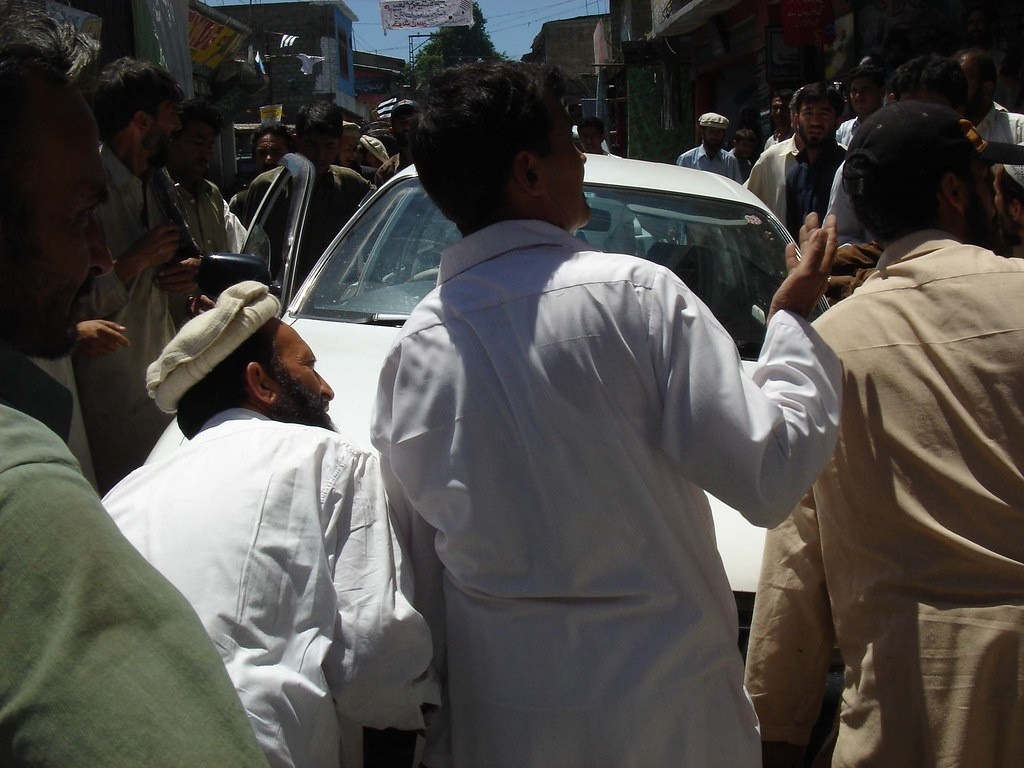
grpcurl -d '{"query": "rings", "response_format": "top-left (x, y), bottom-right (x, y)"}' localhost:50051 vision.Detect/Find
top-left (816, 270), bottom-right (832, 286)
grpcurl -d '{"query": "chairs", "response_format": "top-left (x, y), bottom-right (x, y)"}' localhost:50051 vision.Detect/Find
top-left (645, 242), bottom-right (713, 300)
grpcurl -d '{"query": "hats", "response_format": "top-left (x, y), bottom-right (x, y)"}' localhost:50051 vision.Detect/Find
top-left (343, 120), bottom-right (362, 140)
top-left (698, 113), bottom-right (730, 130)
top-left (842, 99), bottom-right (1024, 196)
top-left (389, 99), bottom-right (422, 121)
top-left (360, 134), bottom-right (390, 163)
top-left (146, 281), bottom-right (280, 414)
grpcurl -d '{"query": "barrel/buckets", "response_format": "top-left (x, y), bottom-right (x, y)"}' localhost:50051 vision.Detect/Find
top-left (260, 104), bottom-right (282, 124)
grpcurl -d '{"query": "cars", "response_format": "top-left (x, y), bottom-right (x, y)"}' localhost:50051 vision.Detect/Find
top-left (194, 151), bottom-right (845, 768)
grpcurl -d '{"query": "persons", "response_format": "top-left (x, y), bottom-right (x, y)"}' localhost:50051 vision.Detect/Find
top-left (0, 0), bottom-right (1024, 768)
